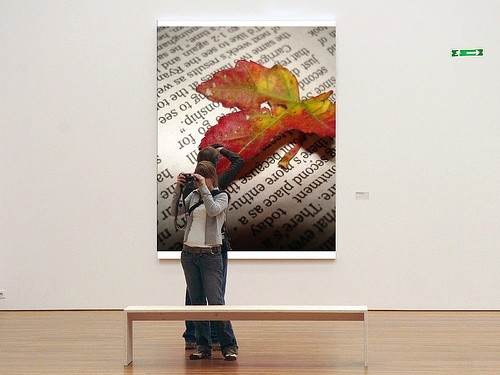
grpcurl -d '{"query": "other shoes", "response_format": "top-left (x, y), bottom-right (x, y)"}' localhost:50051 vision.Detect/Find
top-left (225, 353), bottom-right (237, 361)
top-left (190, 352), bottom-right (213, 359)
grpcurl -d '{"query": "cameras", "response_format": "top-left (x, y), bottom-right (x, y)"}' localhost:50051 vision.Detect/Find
top-left (179, 173), bottom-right (196, 187)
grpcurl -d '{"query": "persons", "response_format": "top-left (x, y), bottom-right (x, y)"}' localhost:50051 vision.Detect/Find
top-left (176, 145), bottom-right (246, 348)
top-left (170, 160), bottom-right (238, 360)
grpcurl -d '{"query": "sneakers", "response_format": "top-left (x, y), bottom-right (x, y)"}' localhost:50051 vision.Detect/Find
top-left (184, 341), bottom-right (196, 349)
top-left (211, 343), bottom-right (222, 350)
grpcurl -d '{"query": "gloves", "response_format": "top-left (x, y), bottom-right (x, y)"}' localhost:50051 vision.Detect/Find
top-left (210, 143), bottom-right (224, 148)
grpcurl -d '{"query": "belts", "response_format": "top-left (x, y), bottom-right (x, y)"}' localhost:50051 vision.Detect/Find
top-left (182, 244), bottom-right (223, 256)
